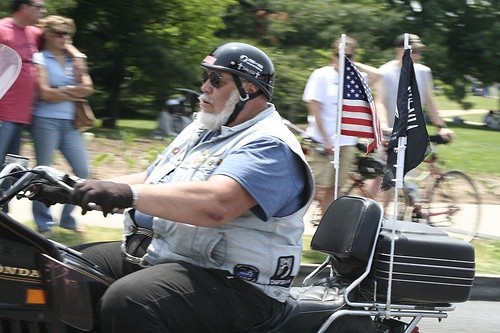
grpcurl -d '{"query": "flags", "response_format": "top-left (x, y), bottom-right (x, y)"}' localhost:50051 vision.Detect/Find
top-left (381, 41), bottom-right (432, 192)
top-left (335, 39), bottom-right (383, 167)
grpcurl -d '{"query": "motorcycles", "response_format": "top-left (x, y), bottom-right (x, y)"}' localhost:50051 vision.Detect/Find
top-left (0, 151), bottom-right (477, 333)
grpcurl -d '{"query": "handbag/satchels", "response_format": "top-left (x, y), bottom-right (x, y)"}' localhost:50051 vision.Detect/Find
top-left (74, 98), bottom-right (98, 130)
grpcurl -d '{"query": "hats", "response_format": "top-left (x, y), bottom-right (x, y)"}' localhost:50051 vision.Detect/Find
top-left (393, 33), bottom-right (426, 49)
top-left (329, 35), bottom-right (360, 55)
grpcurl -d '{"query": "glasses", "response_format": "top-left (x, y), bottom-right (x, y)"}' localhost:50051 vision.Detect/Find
top-left (55, 32), bottom-right (69, 38)
top-left (29, 4), bottom-right (46, 10)
top-left (202, 70), bottom-right (247, 88)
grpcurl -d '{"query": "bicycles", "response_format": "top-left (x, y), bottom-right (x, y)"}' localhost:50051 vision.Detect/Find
top-left (347, 131), bottom-right (481, 246)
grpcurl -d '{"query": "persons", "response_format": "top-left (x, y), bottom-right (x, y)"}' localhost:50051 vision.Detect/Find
top-left (375, 33), bottom-right (455, 218)
top-left (75, 42), bottom-right (316, 333)
top-left (0, 0), bottom-right (93, 235)
top-left (302, 36), bottom-right (385, 219)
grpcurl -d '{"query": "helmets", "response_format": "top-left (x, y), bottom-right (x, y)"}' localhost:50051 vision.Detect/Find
top-left (201, 42), bottom-right (274, 97)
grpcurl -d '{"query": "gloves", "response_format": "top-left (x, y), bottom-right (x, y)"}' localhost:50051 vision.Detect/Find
top-left (67, 180), bottom-right (134, 215)
